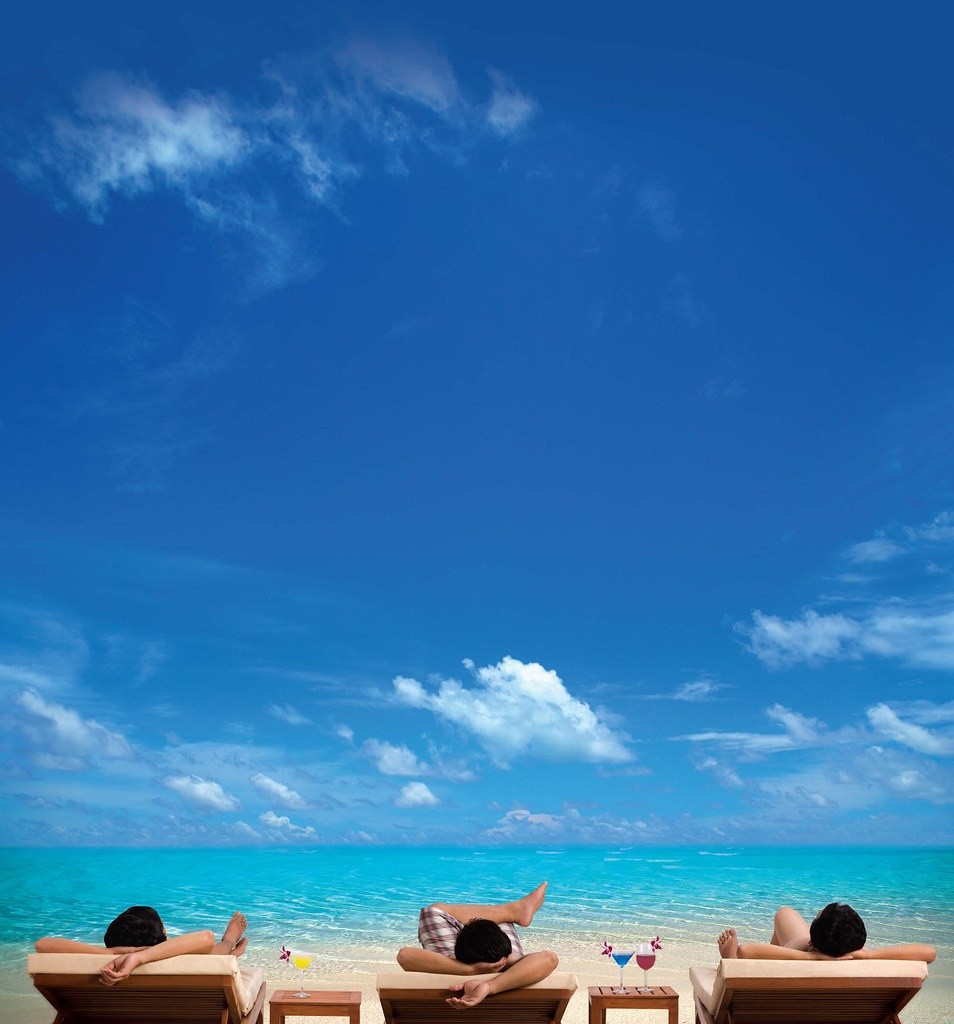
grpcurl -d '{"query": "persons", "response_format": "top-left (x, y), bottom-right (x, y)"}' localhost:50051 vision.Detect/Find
top-left (34, 906), bottom-right (248, 986)
top-left (396, 881), bottom-right (559, 1010)
top-left (718, 903), bottom-right (936, 964)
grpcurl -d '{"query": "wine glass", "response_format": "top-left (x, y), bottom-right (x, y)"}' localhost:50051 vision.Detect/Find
top-left (291, 955), bottom-right (312, 998)
top-left (636, 943), bottom-right (656, 992)
top-left (611, 949), bottom-right (635, 994)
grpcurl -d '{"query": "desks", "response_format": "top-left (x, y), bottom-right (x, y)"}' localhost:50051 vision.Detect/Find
top-left (269, 990), bottom-right (363, 1024)
top-left (587, 986), bottom-right (680, 1024)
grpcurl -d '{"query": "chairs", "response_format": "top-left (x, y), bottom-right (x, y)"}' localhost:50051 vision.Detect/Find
top-left (377, 973), bottom-right (578, 1024)
top-left (28, 954), bottom-right (266, 1024)
top-left (690, 958), bottom-right (929, 1024)
